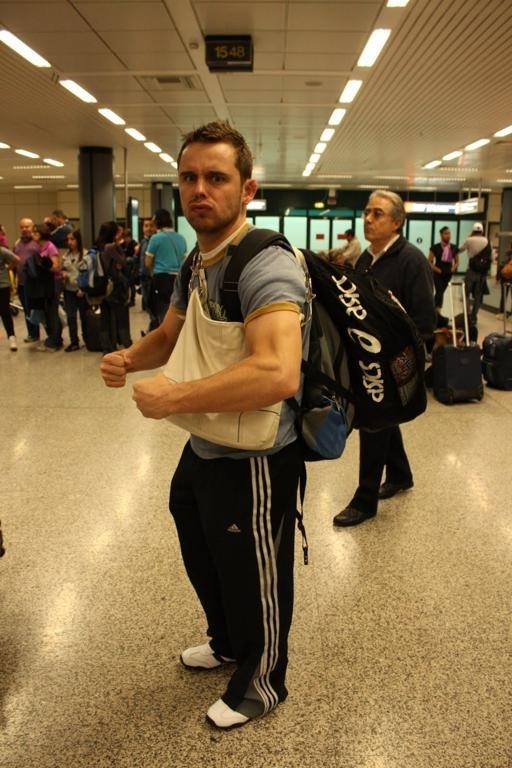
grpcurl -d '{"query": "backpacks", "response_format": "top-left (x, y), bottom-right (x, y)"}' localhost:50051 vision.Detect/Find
top-left (223, 228), bottom-right (427, 462)
top-left (470, 244), bottom-right (491, 275)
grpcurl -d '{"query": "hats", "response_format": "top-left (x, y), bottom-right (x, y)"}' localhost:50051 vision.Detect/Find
top-left (473, 222), bottom-right (483, 232)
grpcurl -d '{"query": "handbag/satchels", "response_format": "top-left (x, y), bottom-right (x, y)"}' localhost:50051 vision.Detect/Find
top-left (427, 313), bottom-right (478, 353)
top-left (77, 248), bottom-right (136, 352)
top-left (163, 242), bottom-right (312, 451)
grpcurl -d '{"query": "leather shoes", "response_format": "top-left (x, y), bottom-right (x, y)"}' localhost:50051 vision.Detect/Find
top-left (334, 506), bottom-right (376, 527)
top-left (378, 481), bottom-right (413, 500)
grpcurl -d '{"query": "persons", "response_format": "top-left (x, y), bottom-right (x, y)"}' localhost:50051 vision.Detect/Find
top-left (428, 226), bottom-right (460, 313)
top-left (333, 191), bottom-right (437, 528)
top-left (319, 230), bottom-right (362, 269)
top-left (99, 117), bottom-right (318, 730)
top-left (0, 209), bottom-right (188, 359)
top-left (458, 223), bottom-right (492, 326)
top-left (495, 250), bottom-right (512, 323)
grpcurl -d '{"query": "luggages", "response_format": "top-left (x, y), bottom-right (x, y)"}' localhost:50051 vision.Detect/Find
top-left (431, 282), bottom-right (483, 406)
top-left (483, 284), bottom-right (512, 390)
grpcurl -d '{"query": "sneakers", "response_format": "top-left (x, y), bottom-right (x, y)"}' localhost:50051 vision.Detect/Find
top-left (206, 699), bottom-right (250, 730)
top-left (25, 336), bottom-right (79, 352)
top-left (9, 336), bottom-right (16, 350)
top-left (180, 641), bottom-right (236, 670)
